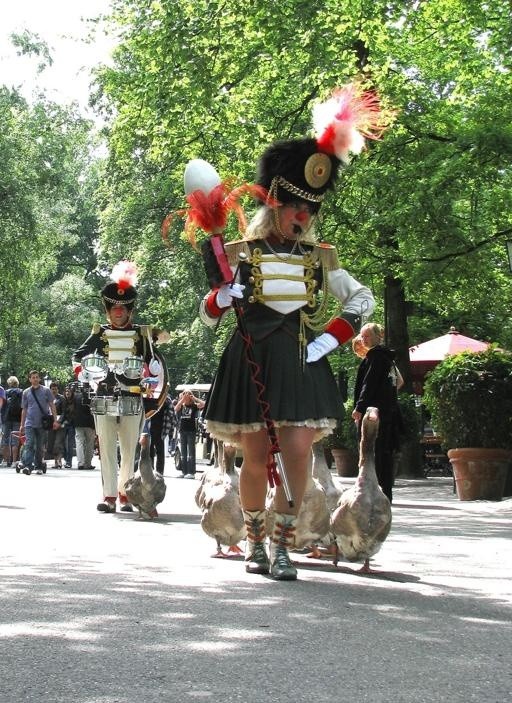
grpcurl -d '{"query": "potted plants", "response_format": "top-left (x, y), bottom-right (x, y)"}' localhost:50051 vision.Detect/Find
top-left (422, 340), bottom-right (512, 501)
top-left (327, 391), bottom-right (421, 477)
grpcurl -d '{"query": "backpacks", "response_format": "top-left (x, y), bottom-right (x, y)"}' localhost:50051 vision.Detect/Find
top-left (3, 390), bottom-right (21, 421)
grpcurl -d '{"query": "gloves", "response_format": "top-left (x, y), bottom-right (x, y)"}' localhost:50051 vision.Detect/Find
top-left (149, 358), bottom-right (161, 376)
top-left (78, 372), bottom-right (88, 383)
top-left (216, 282), bottom-right (246, 309)
top-left (306, 333), bottom-right (340, 363)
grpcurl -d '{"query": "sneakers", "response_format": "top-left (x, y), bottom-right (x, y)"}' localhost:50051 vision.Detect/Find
top-left (174, 470), bottom-right (195, 479)
top-left (97, 497), bottom-right (116, 512)
top-left (119, 492), bottom-right (133, 511)
top-left (0, 454), bottom-right (95, 475)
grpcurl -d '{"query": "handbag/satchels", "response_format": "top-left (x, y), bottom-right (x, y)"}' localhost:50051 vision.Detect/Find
top-left (42, 415), bottom-right (53, 430)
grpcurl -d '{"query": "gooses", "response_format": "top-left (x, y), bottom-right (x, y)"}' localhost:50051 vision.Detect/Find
top-left (312, 437), bottom-right (344, 554)
top-left (267, 446), bottom-right (331, 558)
top-left (328, 406), bottom-right (393, 575)
top-left (119, 432), bottom-right (166, 521)
top-left (195, 438), bottom-right (248, 558)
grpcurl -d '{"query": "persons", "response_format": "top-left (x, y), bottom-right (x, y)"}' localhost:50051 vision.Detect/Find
top-left (198, 139), bottom-right (377, 580)
top-left (351, 322), bottom-right (405, 506)
top-left (71, 283), bottom-right (156, 513)
top-left (0, 369), bottom-right (214, 479)
top-left (352, 333), bottom-right (404, 388)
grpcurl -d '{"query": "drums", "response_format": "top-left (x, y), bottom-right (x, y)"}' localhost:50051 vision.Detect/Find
top-left (145, 343), bottom-right (170, 420)
top-left (90, 394), bottom-right (143, 417)
top-left (82, 354), bottom-right (109, 382)
top-left (123, 355), bottom-right (145, 379)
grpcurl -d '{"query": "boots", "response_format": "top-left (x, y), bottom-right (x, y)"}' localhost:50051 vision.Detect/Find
top-left (241, 508), bottom-right (270, 573)
top-left (270, 512), bottom-right (298, 580)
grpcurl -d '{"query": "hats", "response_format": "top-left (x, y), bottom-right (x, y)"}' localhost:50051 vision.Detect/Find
top-left (259, 138), bottom-right (340, 203)
top-left (102, 283), bottom-right (137, 305)
